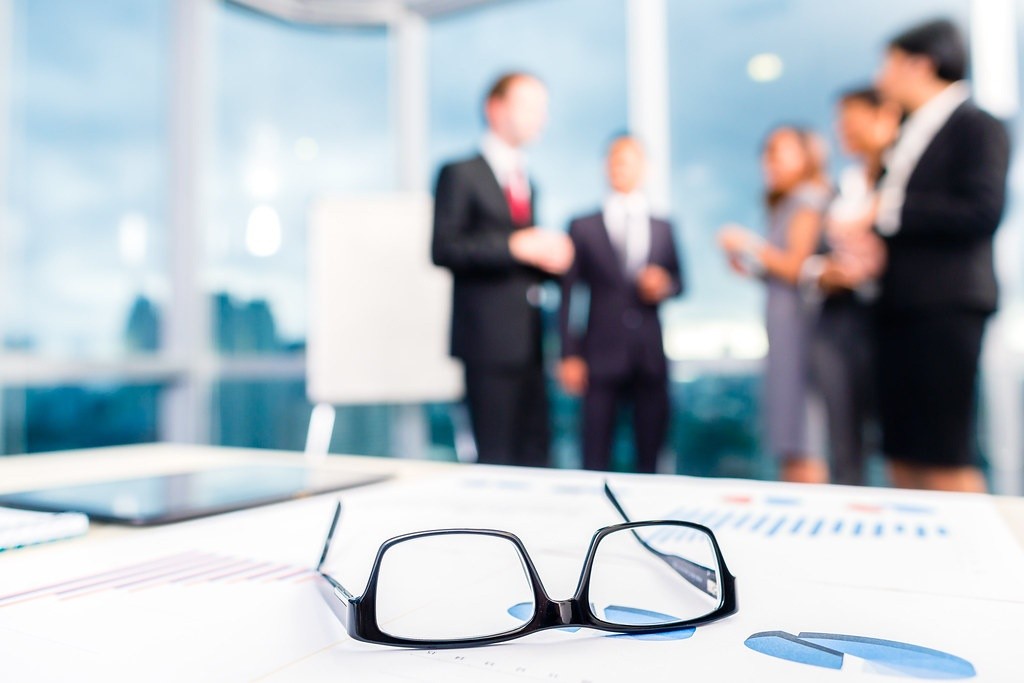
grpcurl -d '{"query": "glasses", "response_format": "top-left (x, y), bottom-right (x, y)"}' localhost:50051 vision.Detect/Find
top-left (317, 484), bottom-right (739, 651)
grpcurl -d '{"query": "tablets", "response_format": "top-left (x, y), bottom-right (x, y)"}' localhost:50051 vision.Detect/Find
top-left (0, 464), bottom-right (394, 527)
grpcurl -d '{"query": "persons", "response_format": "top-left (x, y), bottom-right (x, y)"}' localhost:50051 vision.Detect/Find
top-left (432, 71), bottom-right (575, 466)
top-left (557, 138), bottom-right (686, 475)
top-left (718, 16), bottom-right (1010, 493)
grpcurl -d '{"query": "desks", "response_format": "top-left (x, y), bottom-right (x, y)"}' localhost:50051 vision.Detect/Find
top-left (0, 442), bottom-right (1024, 683)
